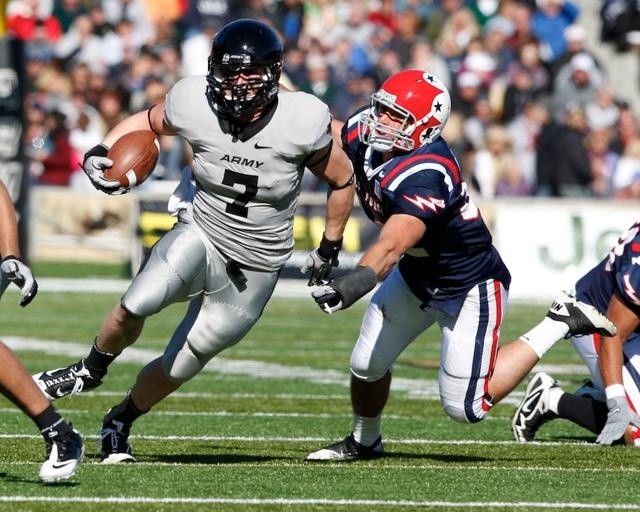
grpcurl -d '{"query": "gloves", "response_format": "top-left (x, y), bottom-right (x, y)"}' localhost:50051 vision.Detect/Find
top-left (300, 232), bottom-right (342, 285)
top-left (0, 253), bottom-right (39, 308)
top-left (81, 142), bottom-right (129, 196)
top-left (595, 381), bottom-right (640, 445)
top-left (308, 283), bottom-right (342, 315)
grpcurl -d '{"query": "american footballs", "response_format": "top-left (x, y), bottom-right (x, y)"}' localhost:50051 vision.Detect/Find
top-left (103, 131), bottom-right (159, 188)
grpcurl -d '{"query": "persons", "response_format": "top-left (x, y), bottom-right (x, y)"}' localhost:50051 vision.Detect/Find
top-left (509, 220), bottom-right (640, 447)
top-left (0, 184), bottom-right (87, 481)
top-left (1, 0), bottom-right (640, 199)
top-left (275, 67), bottom-right (618, 461)
top-left (30, 16), bottom-right (356, 466)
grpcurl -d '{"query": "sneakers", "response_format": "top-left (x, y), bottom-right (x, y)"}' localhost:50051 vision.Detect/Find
top-left (510, 370), bottom-right (562, 443)
top-left (546, 287), bottom-right (618, 340)
top-left (30, 360), bottom-right (104, 401)
top-left (36, 426), bottom-right (88, 484)
top-left (304, 436), bottom-right (387, 465)
top-left (98, 404), bottom-right (139, 464)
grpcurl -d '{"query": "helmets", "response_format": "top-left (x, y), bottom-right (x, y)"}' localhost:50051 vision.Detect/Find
top-left (356, 69), bottom-right (453, 152)
top-left (204, 19), bottom-right (287, 142)
